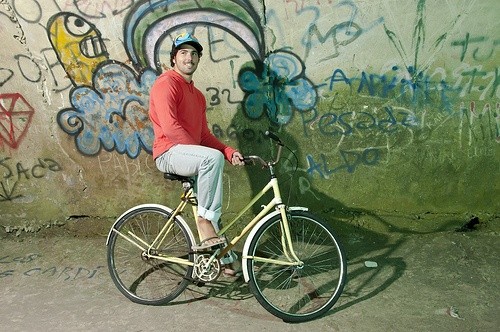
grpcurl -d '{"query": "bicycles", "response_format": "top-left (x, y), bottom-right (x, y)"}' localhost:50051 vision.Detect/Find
top-left (104, 131), bottom-right (348, 323)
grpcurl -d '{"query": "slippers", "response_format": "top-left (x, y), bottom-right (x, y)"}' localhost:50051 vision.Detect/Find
top-left (223, 266), bottom-right (243, 278)
top-left (201, 236), bottom-right (224, 248)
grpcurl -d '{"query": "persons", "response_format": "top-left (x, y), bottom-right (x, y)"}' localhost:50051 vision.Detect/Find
top-left (148, 32), bottom-right (245, 278)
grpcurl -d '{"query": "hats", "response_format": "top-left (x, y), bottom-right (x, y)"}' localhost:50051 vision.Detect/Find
top-left (173, 32), bottom-right (203, 53)
